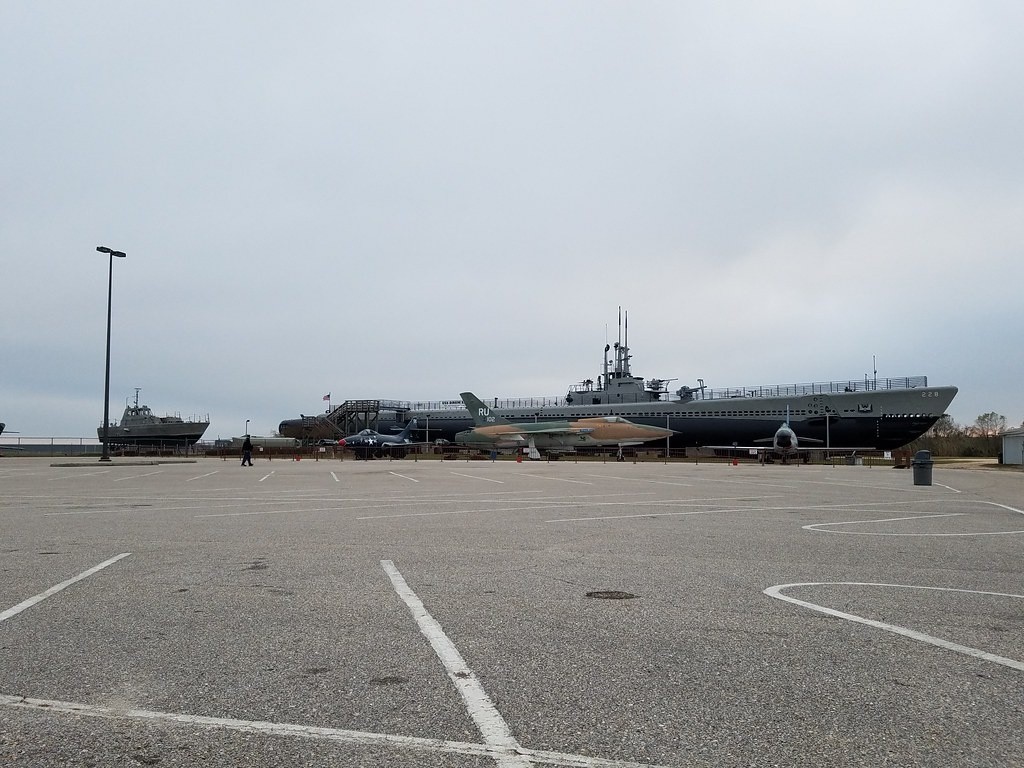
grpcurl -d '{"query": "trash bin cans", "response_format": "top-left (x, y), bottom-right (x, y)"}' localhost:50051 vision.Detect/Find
top-left (911, 461), bottom-right (934, 485)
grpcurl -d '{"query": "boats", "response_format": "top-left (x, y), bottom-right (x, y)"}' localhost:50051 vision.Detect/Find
top-left (203, 417), bottom-right (304, 460)
top-left (96, 384), bottom-right (211, 446)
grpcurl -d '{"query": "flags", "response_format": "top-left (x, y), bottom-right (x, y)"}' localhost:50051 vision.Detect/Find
top-left (323, 395), bottom-right (330, 400)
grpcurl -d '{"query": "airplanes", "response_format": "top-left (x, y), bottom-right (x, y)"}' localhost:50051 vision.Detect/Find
top-left (336, 417), bottom-right (445, 458)
top-left (454, 391), bottom-right (683, 460)
top-left (752, 403), bottom-right (824, 457)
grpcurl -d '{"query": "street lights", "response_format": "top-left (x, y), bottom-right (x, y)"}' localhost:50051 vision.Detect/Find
top-left (97, 246), bottom-right (130, 461)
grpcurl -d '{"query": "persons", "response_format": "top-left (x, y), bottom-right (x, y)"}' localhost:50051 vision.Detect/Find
top-left (240, 438), bottom-right (253, 467)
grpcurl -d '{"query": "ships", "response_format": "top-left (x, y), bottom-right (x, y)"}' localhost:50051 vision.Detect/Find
top-left (278, 305), bottom-right (959, 452)
